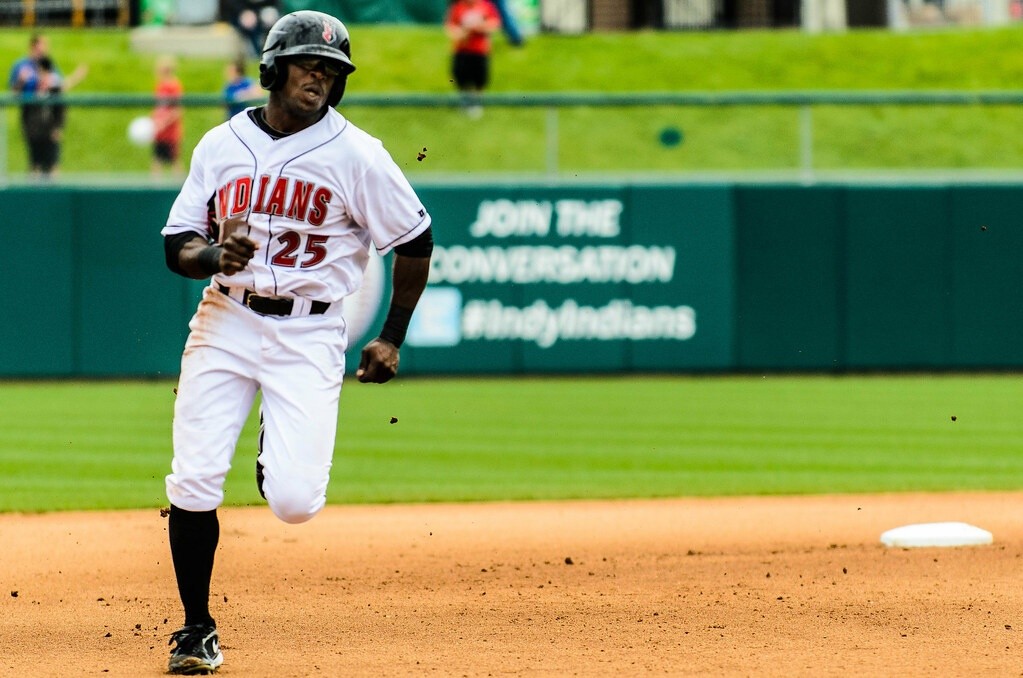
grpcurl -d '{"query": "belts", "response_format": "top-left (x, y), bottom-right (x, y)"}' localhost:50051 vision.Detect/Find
top-left (217, 281), bottom-right (330, 314)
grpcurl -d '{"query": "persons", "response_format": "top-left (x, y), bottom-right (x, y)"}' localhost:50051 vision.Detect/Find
top-left (445, 0), bottom-right (501, 120)
top-left (223, 61), bottom-right (258, 120)
top-left (8, 36), bottom-right (65, 176)
top-left (160, 10), bottom-right (433, 671)
top-left (151, 61), bottom-right (183, 178)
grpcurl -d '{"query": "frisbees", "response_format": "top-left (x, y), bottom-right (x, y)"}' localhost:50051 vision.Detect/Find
top-left (127, 115), bottom-right (157, 145)
top-left (880, 520), bottom-right (995, 549)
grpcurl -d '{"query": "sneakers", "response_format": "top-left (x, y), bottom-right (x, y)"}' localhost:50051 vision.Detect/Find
top-left (168, 623), bottom-right (223, 673)
top-left (255, 401), bottom-right (266, 501)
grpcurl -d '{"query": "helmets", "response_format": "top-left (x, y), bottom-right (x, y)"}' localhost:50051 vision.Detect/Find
top-left (259, 10), bottom-right (355, 109)
